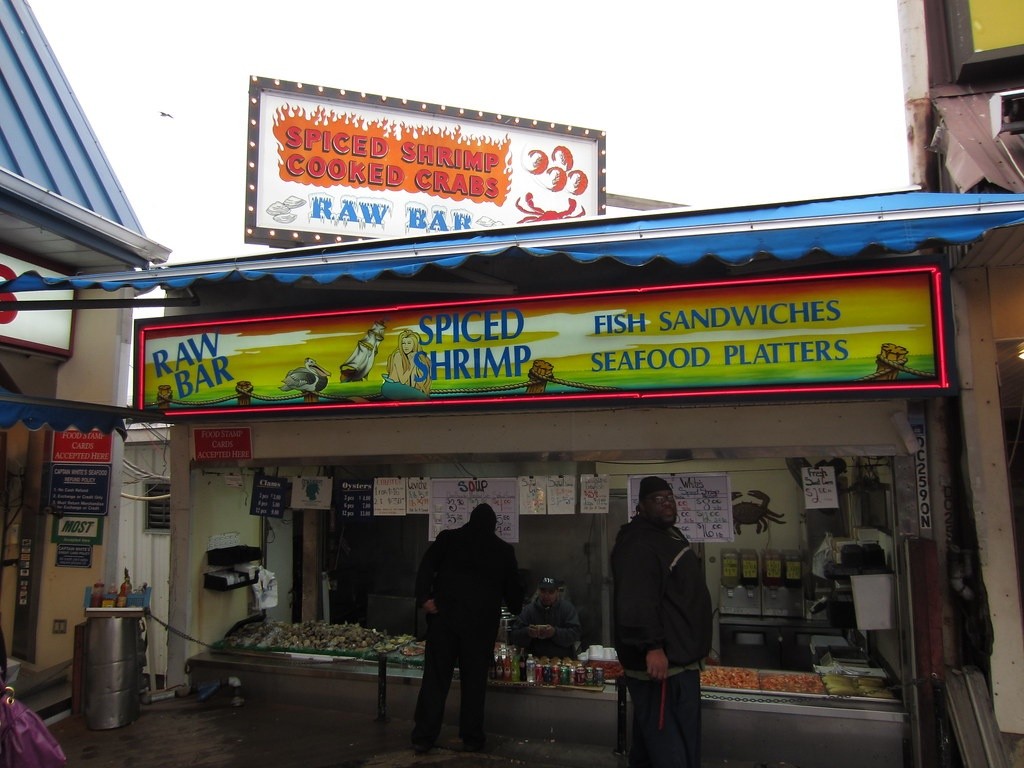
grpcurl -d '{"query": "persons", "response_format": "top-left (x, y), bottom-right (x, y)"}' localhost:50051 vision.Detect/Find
top-left (610, 476), bottom-right (713, 768)
top-left (411, 503), bottom-right (523, 753)
top-left (511, 576), bottom-right (581, 658)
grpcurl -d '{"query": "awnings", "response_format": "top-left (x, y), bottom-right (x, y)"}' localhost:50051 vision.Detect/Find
top-left (0, 192), bottom-right (960, 295)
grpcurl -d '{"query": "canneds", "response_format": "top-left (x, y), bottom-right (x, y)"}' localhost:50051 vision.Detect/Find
top-left (535, 663), bottom-right (604, 687)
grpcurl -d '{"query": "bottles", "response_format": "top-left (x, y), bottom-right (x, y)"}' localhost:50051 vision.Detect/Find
top-left (117, 588), bottom-right (127, 607)
top-left (92, 579), bottom-right (105, 607)
top-left (489, 648), bottom-right (536, 682)
top-left (121, 576), bottom-right (132, 595)
top-left (107, 583), bottom-right (118, 594)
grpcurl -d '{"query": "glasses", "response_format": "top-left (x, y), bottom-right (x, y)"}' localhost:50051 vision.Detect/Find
top-left (642, 493), bottom-right (674, 503)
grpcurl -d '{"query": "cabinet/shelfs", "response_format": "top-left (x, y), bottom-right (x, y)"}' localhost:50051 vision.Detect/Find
top-left (203, 545), bottom-right (263, 592)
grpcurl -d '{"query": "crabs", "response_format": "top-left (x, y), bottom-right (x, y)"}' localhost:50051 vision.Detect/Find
top-left (731, 489), bottom-right (787, 535)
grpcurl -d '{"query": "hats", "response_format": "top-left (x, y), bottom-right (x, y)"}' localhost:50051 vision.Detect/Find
top-left (639, 477), bottom-right (670, 503)
top-left (538, 576), bottom-right (558, 590)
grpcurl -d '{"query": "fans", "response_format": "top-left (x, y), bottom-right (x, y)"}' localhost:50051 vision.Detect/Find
top-left (784, 457), bottom-right (847, 514)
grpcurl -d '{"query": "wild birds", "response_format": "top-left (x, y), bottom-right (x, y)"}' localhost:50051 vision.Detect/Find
top-left (160, 112), bottom-right (173, 118)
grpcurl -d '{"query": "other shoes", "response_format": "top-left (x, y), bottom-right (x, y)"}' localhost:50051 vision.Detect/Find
top-left (414, 742), bottom-right (433, 752)
top-left (464, 733), bottom-right (488, 751)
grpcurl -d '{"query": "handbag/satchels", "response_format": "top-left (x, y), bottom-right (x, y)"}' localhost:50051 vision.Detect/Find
top-left (0, 691), bottom-right (68, 768)
